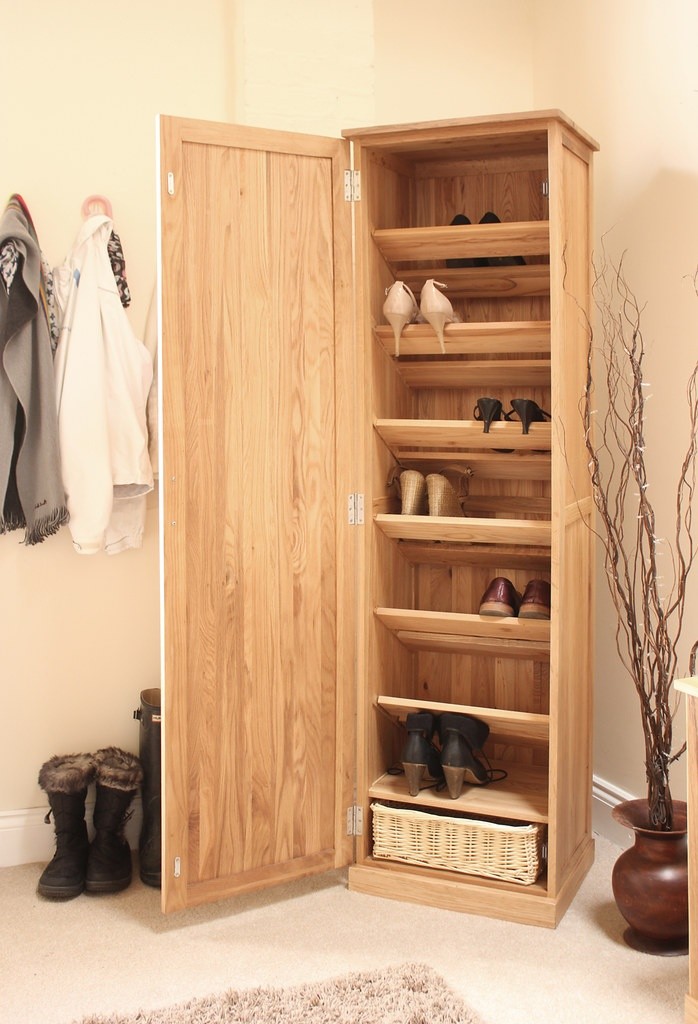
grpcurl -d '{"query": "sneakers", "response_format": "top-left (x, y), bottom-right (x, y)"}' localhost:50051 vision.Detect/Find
top-left (476, 576), bottom-right (518, 618)
top-left (516, 579), bottom-right (550, 618)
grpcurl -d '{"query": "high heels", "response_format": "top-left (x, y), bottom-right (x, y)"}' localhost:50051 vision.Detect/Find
top-left (386, 463), bottom-right (425, 518)
top-left (508, 399), bottom-right (549, 435)
top-left (422, 465), bottom-right (473, 519)
top-left (397, 710), bottom-right (444, 799)
top-left (472, 397), bottom-right (514, 454)
top-left (381, 280), bottom-right (418, 359)
top-left (434, 714), bottom-right (492, 800)
top-left (421, 278), bottom-right (459, 358)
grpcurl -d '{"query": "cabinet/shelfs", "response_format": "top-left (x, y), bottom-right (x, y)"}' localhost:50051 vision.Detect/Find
top-left (158, 108), bottom-right (600, 931)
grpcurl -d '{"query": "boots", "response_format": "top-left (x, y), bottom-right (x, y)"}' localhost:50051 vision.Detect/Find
top-left (84, 745), bottom-right (145, 895)
top-left (132, 687), bottom-right (163, 888)
top-left (32, 751), bottom-right (95, 900)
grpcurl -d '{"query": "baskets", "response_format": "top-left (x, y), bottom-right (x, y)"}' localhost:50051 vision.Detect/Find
top-left (368, 798), bottom-right (549, 887)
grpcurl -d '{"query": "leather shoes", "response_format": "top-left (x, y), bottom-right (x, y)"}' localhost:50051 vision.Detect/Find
top-left (478, 213), bottom-right (525, 268)
top-left (443, 215), bottom-right (482, 268)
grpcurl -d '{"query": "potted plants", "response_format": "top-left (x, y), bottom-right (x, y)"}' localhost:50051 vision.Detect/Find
top-left (556, 230), bottom-right (696, 945)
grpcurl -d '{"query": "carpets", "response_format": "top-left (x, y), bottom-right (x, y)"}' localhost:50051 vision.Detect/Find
top-left (54, 960), bottom-right (485, 1022)
